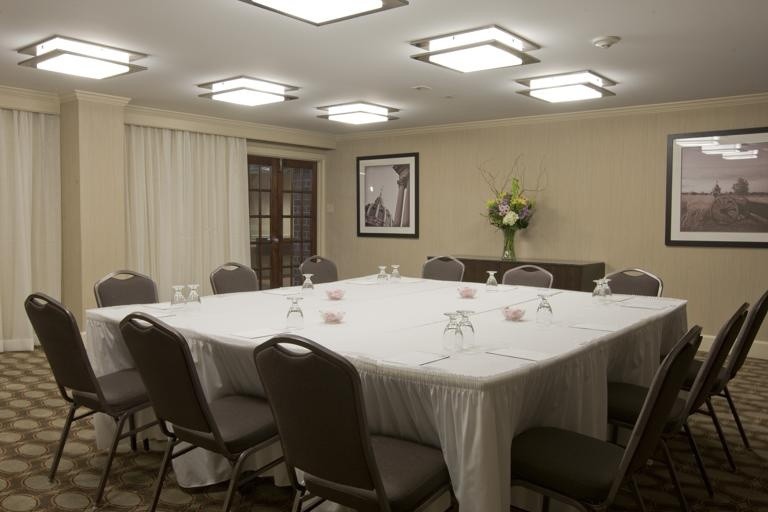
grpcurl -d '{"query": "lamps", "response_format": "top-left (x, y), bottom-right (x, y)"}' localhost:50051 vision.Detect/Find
top-left (513, 68), bottom-right (619, 105)
top-left (238, 0), bottom-right (409, 28)
top-left (313, 100), bottom-right (401, 126)
top-left (406, 23), bottom-right (543, 74)
top-left (16, 34), bottom-right (149, 82)
top-left (195, 73), bottom-right (300, 109)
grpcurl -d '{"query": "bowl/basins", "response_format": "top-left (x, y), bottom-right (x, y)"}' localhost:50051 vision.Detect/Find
top-left (458, 285), bottom-right (477, 298)
top-left (326, 286), bottom-right (345, 301)
top-left (500, 304), bottom-right (525, 322)
top-left (313, 311), bottom-right (346, 324)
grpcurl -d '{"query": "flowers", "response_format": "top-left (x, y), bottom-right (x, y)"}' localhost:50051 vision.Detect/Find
top-left (469, 149), bottom-right (551, 260)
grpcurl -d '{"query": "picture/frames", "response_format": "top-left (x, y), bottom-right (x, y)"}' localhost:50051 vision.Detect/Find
top-left (663, 126), bottom-right (768, 249)
top-left (354, 151), bottom-right (420, 240)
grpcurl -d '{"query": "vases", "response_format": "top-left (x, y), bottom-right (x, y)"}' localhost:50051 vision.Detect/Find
top-left (497, 225), bottom-right (518, 261)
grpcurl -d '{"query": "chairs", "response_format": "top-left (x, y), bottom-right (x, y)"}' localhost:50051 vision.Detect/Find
top-left (605, 300), bottom-right (751, 512)
top-left (297, 254), bottom-right (339, 286)
top-left (418, 253), bottom-right (664, 299)
top-left (655, 289), bottom-right (768, 472)
top-left (209, 261), bottom-right (260, 295)
top-left (505, 321), bottom-right (704, 511)
top-left (252, 332), bottom-right (460, 512)
top-left (20, 290), bottom-right (167, 511)
top-left (117, 309), bottom-right (288, 511)
top-left (92, 268), bottom-right (162, 454)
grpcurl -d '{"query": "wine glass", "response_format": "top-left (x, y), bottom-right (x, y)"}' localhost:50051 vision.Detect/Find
top-left (485, 270), bottom-right (499, 292)
top-left (534, 294), bottom-right (553, 324)
top-left (285, 296), bottom-right (305, 330)
top-left (592, 279), bottom-right (612, 302)
top-left (376, 265), bottom-right (400, 282)
top-left (171, 284), bottom-right (202, 306)
top-left (442, 310), bottom-right (475, 346)
top-left (300, 274), bottom-right (315, 293)
